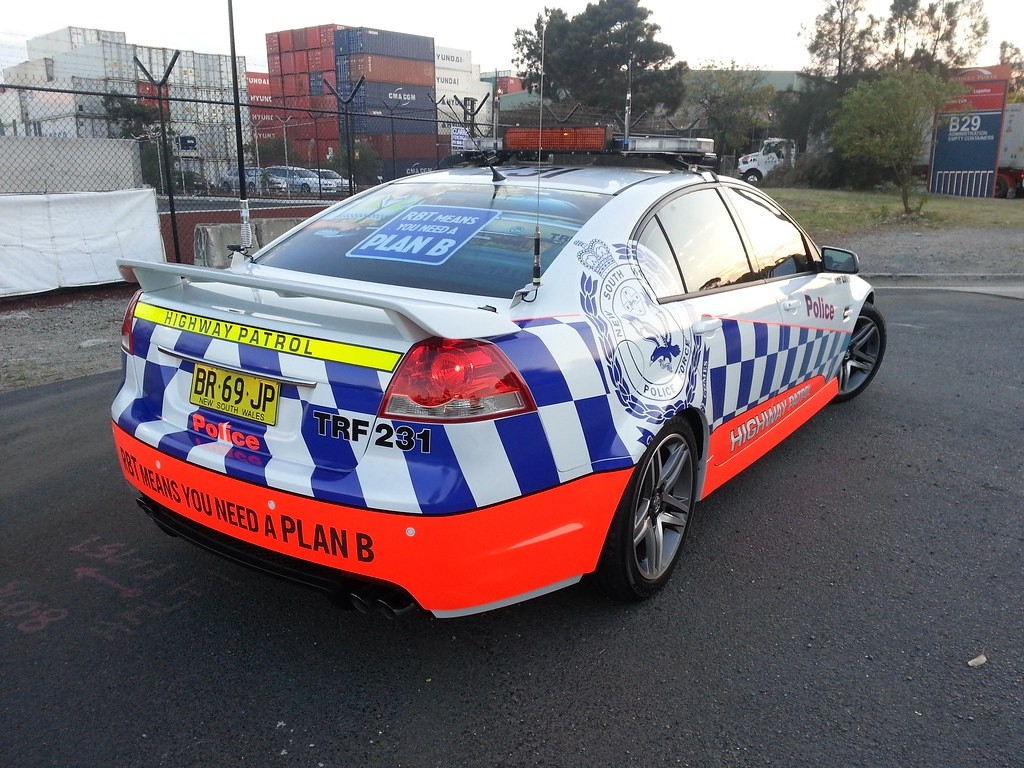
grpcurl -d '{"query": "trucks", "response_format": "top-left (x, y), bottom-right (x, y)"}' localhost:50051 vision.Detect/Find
top-left (735, 139), bottom-right (803, 185)
top-left (995, 102), bottom-right (1024, 199)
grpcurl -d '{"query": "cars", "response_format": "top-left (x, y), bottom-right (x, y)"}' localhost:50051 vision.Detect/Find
top-left (221, 165), bottom-right (285, 195)
top-left (177, 170), bottom-right (214, 189)
top-left (309, 168), bottom-right (356, 193)
top-left (107, 164), bottom-right (887, 629)
top-left (265, 165), bottom-right (337, 196)
